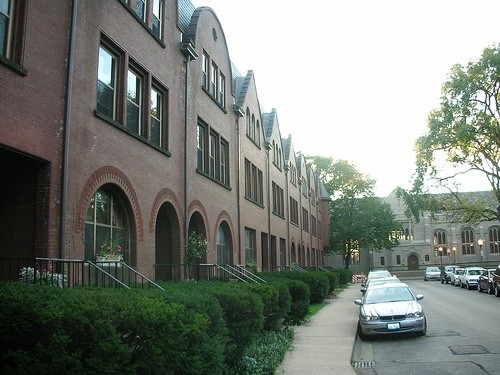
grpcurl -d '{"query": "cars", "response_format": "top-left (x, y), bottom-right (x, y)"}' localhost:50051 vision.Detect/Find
top-left (360, 269), bottom-right (402, 297)
top-left (450, 267), bottom-right (466, 287)
top-left (477, 269), bottom-right (497, 295)
top-left (354, 283), bottom-right (427, 341)
top-left (423, 266), bottom-right (442, 281)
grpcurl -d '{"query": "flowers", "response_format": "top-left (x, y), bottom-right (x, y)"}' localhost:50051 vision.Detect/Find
top-left (96, 233), bottom-right (128, 259)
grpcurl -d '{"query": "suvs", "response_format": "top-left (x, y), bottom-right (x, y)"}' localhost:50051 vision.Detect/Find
top-left (492, 264), bottom-right (500, 297)
top-left (460, 266), bottom-right (487, 290)
top-left (440, 265), bottom-right (461, 284)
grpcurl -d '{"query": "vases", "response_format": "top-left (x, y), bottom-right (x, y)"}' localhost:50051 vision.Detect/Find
top-left (95, 255), bottom-right (121, 262)
